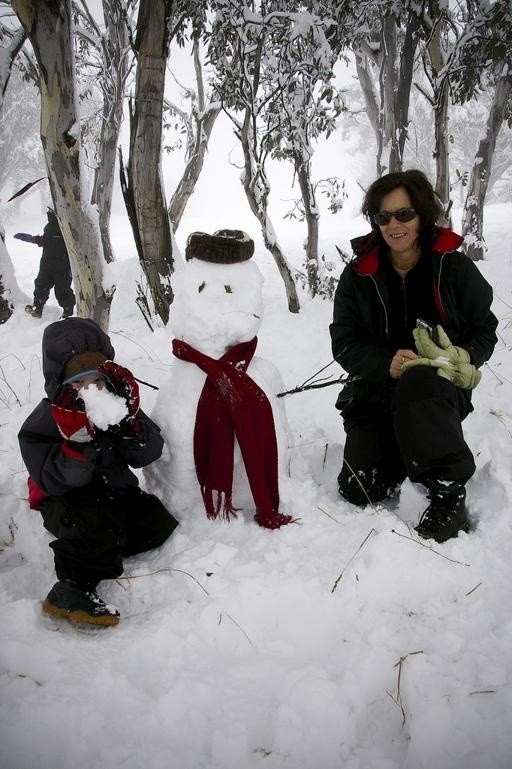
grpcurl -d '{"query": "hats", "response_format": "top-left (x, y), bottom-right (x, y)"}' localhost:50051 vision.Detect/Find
top-left (61, 353), bottom-right (110, 388)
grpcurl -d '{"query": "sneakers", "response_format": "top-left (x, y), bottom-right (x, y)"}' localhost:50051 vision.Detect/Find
top-left (59, 310), bottom-right (73, 321)
top-left (416, 476), bottom-right (469, 541)
top-left (24, 304), bottom-right (42, 318)
top-left (43, 580), bottom-right (119, 625)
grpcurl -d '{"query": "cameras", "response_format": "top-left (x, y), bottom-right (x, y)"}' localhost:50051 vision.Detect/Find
top-left (416, 317), bottom-right (438, 340)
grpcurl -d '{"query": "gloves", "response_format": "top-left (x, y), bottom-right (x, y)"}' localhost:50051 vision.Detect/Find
top-left (95, 360), bottom-right (139, 418)
top-left (49, 382), bottom-right (96, 447)
top-left (412, 323), bottom-right (472, 362)
top-left (399, 358), bottom-right (482, 389)
top-left (13, 233), bottom-right (36, 244)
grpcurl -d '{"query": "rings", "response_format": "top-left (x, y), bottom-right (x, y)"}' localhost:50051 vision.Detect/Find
top-left (400, 364), bottom-right (403, 371)
top-left (400, 358), bottom-right (405, 363)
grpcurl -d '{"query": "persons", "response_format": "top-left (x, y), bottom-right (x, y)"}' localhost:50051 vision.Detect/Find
top-left (12, 199), bottom-right (77, 320)
top-left (18, 316), bottom-right (182, 629)
top-left (326, 169), bottom-right (500, 544)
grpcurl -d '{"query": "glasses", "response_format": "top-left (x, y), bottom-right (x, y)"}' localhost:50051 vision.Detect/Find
top-left (372, 208), bottom-right (418, 227)
